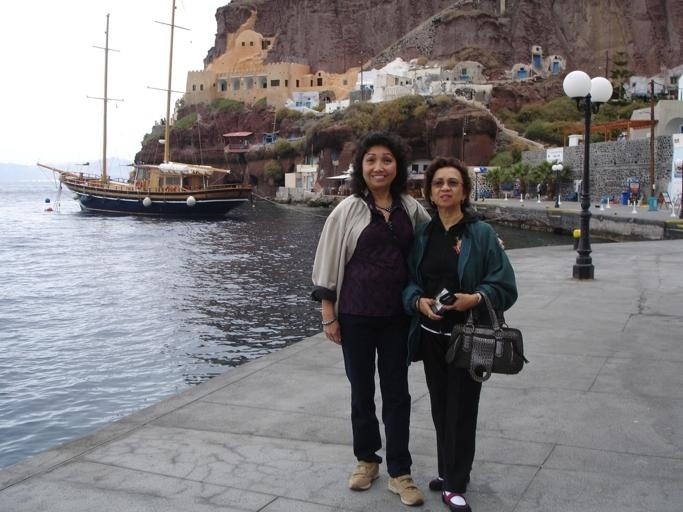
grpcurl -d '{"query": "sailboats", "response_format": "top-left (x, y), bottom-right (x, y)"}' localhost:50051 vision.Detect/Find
top-left (35, 0), bottom-right (255, 220)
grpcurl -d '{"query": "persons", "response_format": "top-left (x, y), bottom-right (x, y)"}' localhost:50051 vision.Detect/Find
top-left (398, 155), bottom-right (519, 512)
top-left (309, 132), bottom-right (432, 507)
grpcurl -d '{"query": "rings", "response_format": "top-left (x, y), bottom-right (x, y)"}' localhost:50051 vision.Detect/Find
top-left (428, 314), bottom-right (431, 318)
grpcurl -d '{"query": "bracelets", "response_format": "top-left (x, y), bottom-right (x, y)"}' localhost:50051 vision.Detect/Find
top-left (321, 317), bottom-right (337, 325)
top-left (477, 292), bottom-right (484, 304)
top-left (416, 296), bottom-right (420, 313)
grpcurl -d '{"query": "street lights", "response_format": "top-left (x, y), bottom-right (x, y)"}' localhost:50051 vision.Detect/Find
top-left (560, 70), bottom-right (613, 279)
top-left (472, 167), bottom-right (482, 202)
top-left (551, 163), bottom-right (564, 208)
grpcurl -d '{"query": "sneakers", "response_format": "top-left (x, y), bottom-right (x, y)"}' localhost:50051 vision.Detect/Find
top-left (349, 461), bottom-right (379, 490)
top-left (388, 475), bottom-right (424, 505)
top-left (430, 478), bottom-right (444, 490)
top-left (442, 489), bottom-right (471, 512)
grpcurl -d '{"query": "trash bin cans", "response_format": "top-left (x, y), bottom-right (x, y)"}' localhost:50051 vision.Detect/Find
top-left (620, 191), bottom-right (629, 205)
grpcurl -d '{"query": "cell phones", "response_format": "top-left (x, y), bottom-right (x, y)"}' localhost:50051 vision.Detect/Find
top-left (438, 291), bottom-right (456, 305)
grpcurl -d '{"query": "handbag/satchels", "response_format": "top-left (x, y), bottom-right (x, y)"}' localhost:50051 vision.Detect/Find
top-left (445, 324), bottom-right (523, 382)
